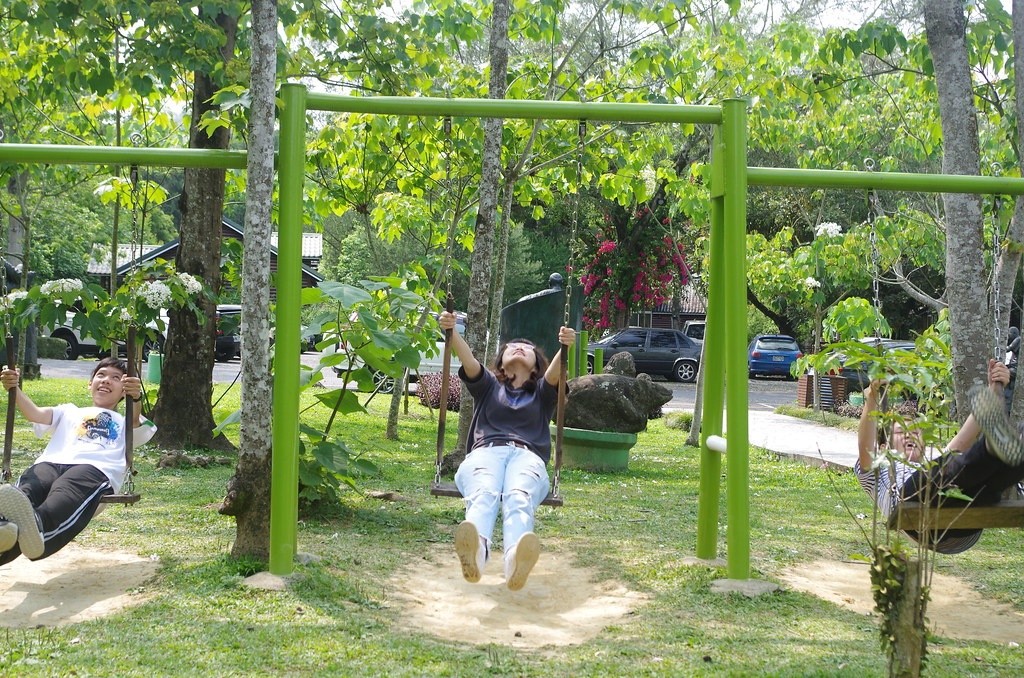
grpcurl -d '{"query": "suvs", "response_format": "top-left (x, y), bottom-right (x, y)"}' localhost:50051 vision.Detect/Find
top-left (322, 307), bottom-right (500, 395)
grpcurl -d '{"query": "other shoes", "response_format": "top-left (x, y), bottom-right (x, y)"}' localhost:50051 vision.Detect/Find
top-left (968, 384), bottom-right (1024, 467)
top-left (455, 521), bottom-right (482, 583)
top-left (0, 483), bottom-right (45, 559)
top-left (504, 532), bottom-right (540, 592)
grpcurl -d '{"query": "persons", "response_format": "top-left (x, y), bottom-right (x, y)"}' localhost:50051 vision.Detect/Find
top-left (1, 357), bottom-right (158, 570)
top-left (438, 310), bottom-right (576, 592)
top-left (853, 356), bottom-right (1024, 554)
top-left (1005, 327), bottom-right (1021, 370)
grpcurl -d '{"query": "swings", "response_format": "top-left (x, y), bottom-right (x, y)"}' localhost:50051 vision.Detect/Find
top-left (426, 111), bottom-right (588, 508)
top-left (0, 147), bottom-right (148, 508)
top-left (861, 161), bottom-right (1024, 534)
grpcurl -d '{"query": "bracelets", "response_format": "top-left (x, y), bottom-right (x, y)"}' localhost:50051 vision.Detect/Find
top-left (133, 388), bottom-right (143, 402)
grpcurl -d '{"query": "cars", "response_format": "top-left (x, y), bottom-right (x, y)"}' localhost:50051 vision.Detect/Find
top-left (214, 304), bottom-right (241, 362)
top-left (682, 320), bottom-right (705, 344)
top-left (587, 326), bottom-right (703, 383)
top-left (819, 340), bottom-right (915, 395)
top-left (44, 296), bottom-right (170, 362)
top-left (269, 325), bottom-right (315, 353)
top-left (748, 334), bottom-right (803, 381)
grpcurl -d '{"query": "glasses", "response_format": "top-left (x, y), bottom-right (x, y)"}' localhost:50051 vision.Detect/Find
top-left (506, 343), bottom-right (534, 351)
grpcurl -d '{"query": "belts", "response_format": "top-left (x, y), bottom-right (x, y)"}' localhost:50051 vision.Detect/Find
top-left (476, 440), bottom-right (528, 450)
